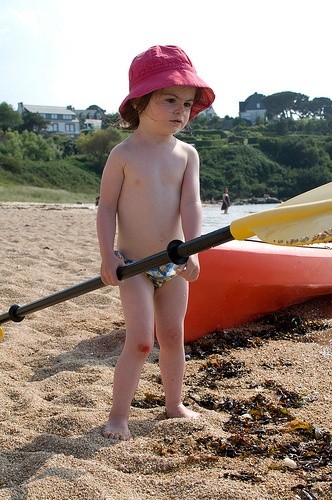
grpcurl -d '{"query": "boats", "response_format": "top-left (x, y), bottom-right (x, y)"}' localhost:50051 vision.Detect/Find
top-left (156, 182), bottom-right (332, 345)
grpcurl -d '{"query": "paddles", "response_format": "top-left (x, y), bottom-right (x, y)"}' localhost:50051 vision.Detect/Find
top-left (0, 181), bottom-right (332, 339)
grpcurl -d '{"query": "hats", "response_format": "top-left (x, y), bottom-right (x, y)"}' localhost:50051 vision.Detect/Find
top-left (119, 45), bottom-right (216, 121)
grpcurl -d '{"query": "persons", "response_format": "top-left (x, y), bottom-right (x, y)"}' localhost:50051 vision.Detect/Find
top-left (92, 43), bottom-right (204, 441)
top-left (221, 187), bottom-right (230, 214)
